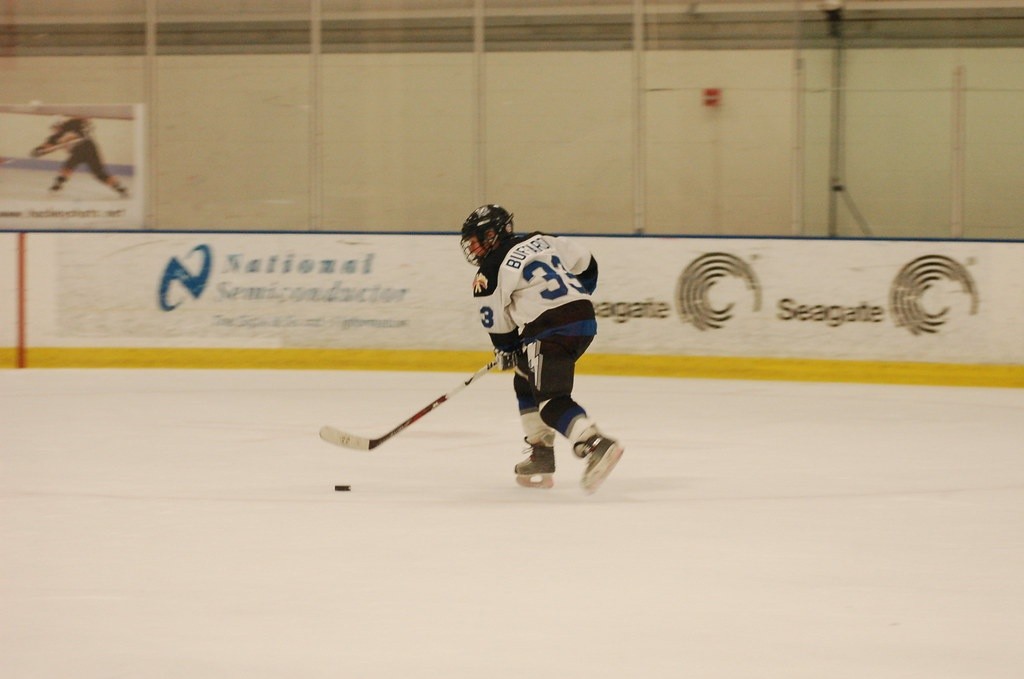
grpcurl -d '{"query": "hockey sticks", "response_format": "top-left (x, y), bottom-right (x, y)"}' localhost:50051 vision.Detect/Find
top-left (317, 360), bottom-right (499, 452)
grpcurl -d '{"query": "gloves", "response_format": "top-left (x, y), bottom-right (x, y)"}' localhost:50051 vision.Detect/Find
top-left (494, 348), bottom-right (519, 370)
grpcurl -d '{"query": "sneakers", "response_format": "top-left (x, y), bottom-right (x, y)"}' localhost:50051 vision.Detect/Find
top-left (515, 432), bottom-right (555, 489)
top-left (573, 426), bottom-right (624, 489)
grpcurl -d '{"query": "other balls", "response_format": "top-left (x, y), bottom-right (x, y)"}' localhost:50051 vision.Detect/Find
top-left (334, 483), bottom-right (351, 491)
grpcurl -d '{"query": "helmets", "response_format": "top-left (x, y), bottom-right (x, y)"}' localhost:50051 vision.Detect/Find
top-left (460, 204), bottom-right (515, 266)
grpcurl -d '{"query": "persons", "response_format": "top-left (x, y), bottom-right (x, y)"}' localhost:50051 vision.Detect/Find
top-left (459, 203), bottom-right (626, 496)
top-left (32, 116), bottom-right (129, 197)
